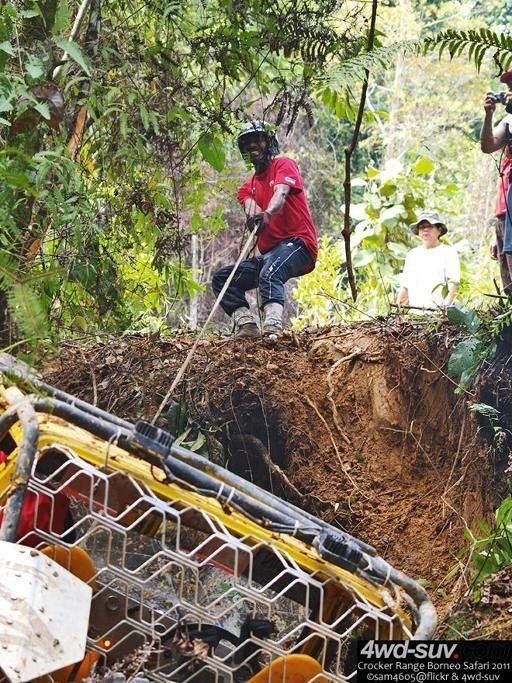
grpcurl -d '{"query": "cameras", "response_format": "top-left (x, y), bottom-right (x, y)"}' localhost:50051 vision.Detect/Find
top-left (493, 94), bottom-right (505, 103)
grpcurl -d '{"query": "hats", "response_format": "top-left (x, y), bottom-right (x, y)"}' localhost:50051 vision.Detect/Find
top-left (409, 212), bottom-right (447, 237)
top-left (501, 68), bottom-right (512, 82)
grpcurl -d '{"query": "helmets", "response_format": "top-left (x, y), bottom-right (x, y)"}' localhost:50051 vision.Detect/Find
top-left (237, 119), bottom-right (275, 137)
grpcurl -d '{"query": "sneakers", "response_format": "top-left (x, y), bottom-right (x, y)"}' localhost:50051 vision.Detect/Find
top-left (262, 326), bottom-right (282, 337)
top-left (230, 323), bottom-right (261, 338)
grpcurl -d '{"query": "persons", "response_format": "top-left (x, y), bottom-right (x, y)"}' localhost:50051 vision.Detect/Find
top-left (489, 120), bottom-right (512, 306)
top-left (399, 210), bottom-right (460, 315)
top-left (480, 69), bottom-right (512, 281)
top-left (212, 119), bottom-right (318, 342)
